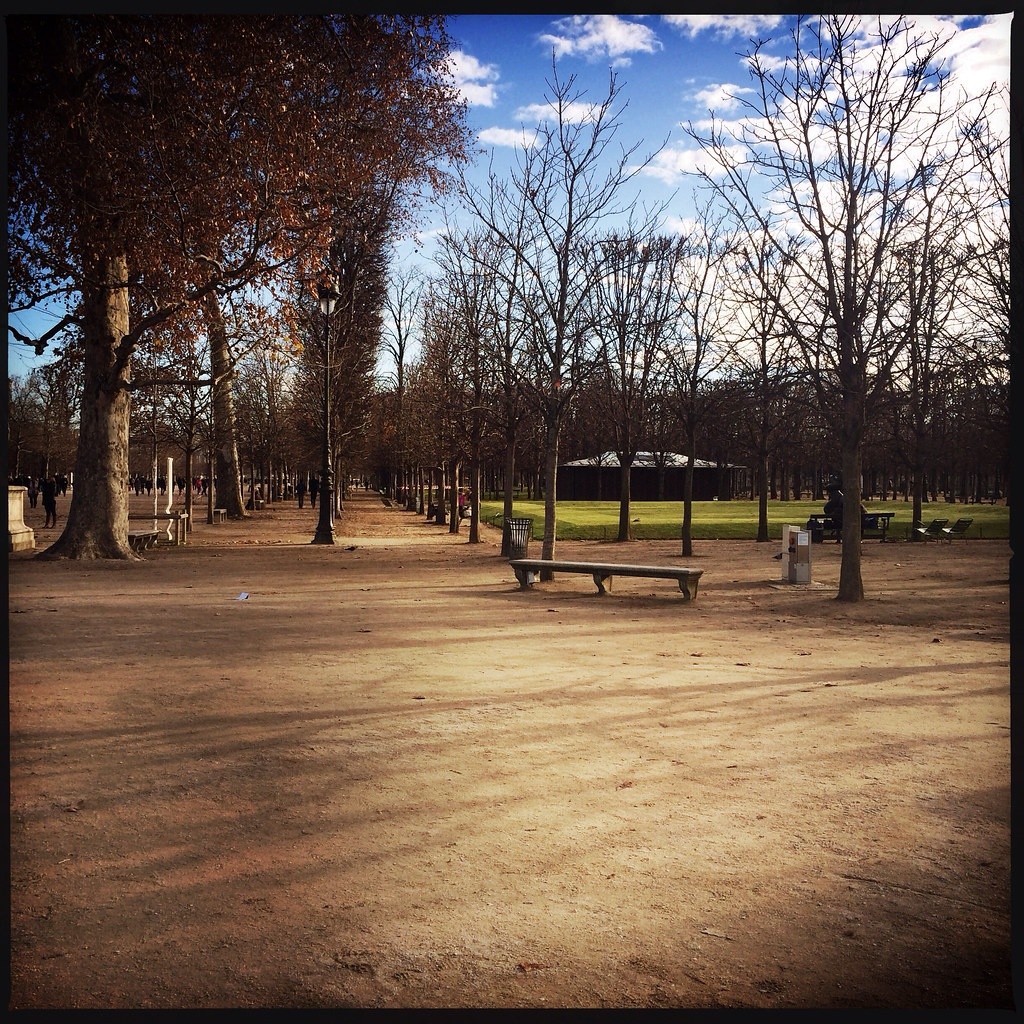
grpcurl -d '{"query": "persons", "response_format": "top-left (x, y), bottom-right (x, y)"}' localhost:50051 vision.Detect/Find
top-left (246, 487), bottom-right (258, 509)
top-left (8, 471), bottom-right (67, 529)
top-left (157, 476), bottom-right (167, 495)
top-left (821, 483), bottom-right (843, 529)
top-left (365, 479), bottom-right (369, 491)
top-left (244, 475), bottom-right (251, 491)
top-left (861, 502), bottom-right (870, 544)
top-left (311, 473), bottom-right (318, 507)
top-left (178, 476), bottom-right (186, 495)
top-left (458, 487), bottom-right (472, 518)
top-left (355, 480), bottom-right (358, 492)
top-left (192, 475), bottom-right (209, 496)
top-left (128, 474), bottom-right (153, 496)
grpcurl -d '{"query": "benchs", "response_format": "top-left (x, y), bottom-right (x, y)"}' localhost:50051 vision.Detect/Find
top-left (803, 512), bottom-right (896, 544)
top-left (254, 499), bottom-right (264, 510)
top-left (127, 530), bottom-right (160, 554)
top-left (213, 509), bottom-right (228, 523)
top-left (509, 558), bottom-right (704, 600)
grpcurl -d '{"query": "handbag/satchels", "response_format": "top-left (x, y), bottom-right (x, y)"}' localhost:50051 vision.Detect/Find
top-left (807, 519), bottom-right (824, 544)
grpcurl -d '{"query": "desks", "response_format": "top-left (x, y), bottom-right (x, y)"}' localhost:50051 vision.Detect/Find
top-left (129, 508), bottom-right (188, 545)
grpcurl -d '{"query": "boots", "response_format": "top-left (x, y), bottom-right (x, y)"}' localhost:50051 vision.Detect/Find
top-left (50, 516), bottom-right (56, 529)
top-left (42, 516), bottom-right (50, 529)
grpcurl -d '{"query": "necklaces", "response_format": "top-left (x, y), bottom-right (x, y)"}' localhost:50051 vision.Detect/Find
top-left (296, 479), bottom-right (305, 508)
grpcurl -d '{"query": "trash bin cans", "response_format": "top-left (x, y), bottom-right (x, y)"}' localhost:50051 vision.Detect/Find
top-left (505, 517), bottom-right (533, 560)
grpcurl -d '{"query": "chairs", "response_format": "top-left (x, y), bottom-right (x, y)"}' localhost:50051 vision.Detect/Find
top-left (910, 518), bottom-right (949, 545)
top-left (940, 518), bottom-right (974, 545)
